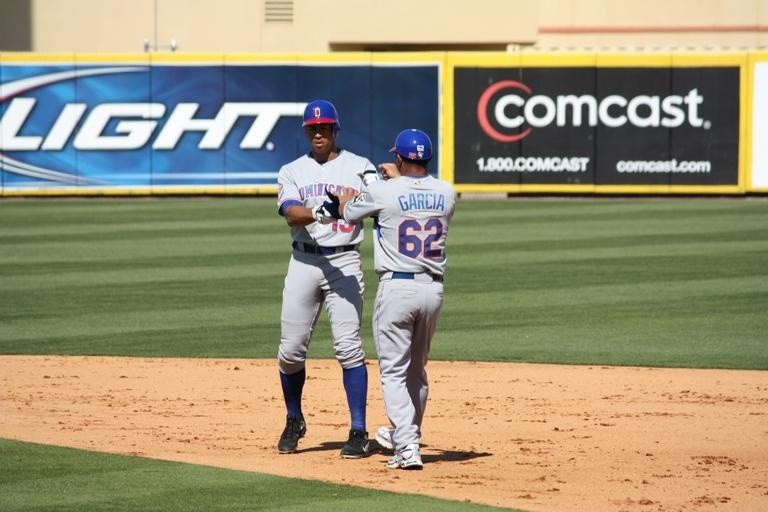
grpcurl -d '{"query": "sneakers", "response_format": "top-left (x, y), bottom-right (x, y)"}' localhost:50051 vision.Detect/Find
top-left (278, 414), bottom-right (306, 454)
top-left (385, 447), bottom-right (423, 468)
top-left (374, 426), bottom-right (394, 450)
top-left (340, 429), bottom-right (370, 458)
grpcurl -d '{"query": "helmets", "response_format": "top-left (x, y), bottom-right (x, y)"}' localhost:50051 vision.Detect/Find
top-left (301, 100), bottom-right (341, 130)
top-left (387, 129), bottom-right (432, 161)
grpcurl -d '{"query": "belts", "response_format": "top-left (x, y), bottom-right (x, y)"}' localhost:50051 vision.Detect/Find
top-left (379, 272), bottom-right (443, 282)
top-left (291, 240), bottom-right (354, 254)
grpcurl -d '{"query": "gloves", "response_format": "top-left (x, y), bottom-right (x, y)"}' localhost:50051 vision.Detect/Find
top-left (311, 204), bottom-right (336, 224)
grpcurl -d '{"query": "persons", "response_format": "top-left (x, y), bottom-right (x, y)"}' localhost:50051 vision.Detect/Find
top-left (276, 100), bottom-right (382, 458)
top-left (335, 129), bottom-right (458, 470)
